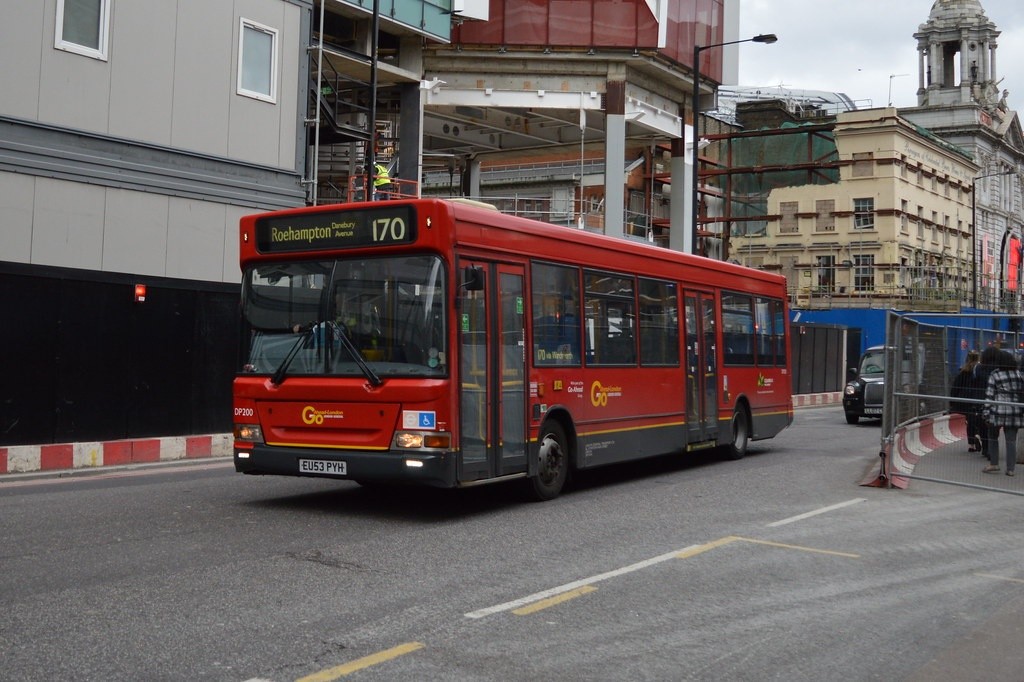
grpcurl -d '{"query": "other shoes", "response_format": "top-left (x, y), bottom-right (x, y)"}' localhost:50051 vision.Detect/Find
top-left (1005, 471), bottom-right (1014, 477)
top-left (975, 435), bottom-right (981, 452)
top-left (968, 447), bottom-right (976, 452)
top-left (982, 467), bottom-right (1000, 473)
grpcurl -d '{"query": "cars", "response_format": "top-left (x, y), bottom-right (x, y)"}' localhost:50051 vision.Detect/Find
top-left (842, 345), bottom-right (927, 425)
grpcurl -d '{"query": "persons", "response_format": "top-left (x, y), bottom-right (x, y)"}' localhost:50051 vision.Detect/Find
top-left (949, 347), bottom-right (1024, 476)
top-left (371, 161), bottom-right (391, 201)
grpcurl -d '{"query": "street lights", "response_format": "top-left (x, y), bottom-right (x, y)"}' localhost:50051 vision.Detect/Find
top-left (692, 34), bottom-right (778, 252)
top-left (972, 166), bottom-right (1020, 348)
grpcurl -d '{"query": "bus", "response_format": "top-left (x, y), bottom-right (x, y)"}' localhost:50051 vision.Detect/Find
top-left (231, 197), bottom-right (794, 500)
